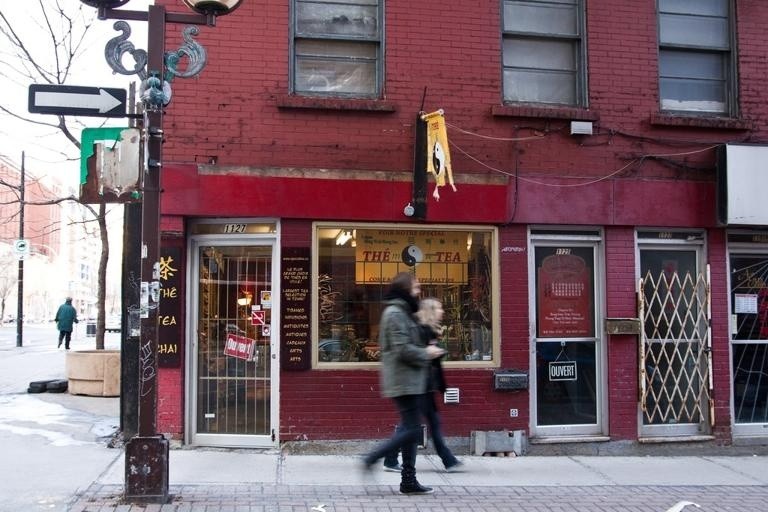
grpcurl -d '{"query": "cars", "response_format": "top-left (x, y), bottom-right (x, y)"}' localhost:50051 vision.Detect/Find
top-left (1, 309), bottom-right (98, 326)
top-left (442, 324), bottom-right (494, 360)
top-left (317, 339), bottom-right (363, 362)
top-left (102, 315), bottom-right (122, 332)
top-left (189, 319), bottom-right (252, 410)
top-left (535, 341), bottom-right (665, 402)
top-left (731, 374), bottom-right (766, 405)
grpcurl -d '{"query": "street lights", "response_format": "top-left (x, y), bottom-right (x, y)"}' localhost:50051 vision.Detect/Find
top-left (85, 1), bottom-right (247, 503)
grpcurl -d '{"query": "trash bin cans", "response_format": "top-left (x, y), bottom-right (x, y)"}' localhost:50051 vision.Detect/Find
top-left (86, 318), bottom-right (96, 337)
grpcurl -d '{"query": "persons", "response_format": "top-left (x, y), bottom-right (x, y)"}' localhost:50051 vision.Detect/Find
top-left (54, 297), bottom-right (79, 350)
top-left (359, 273), bottom-right (446, 492)
top-left (381, 298), bottom-right (471, 473)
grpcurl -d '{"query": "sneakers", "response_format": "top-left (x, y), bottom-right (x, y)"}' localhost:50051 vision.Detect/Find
top-left (400, 482), bottom-right (434, 494)
top-left (383, 463), bottom-right (403, 472)
